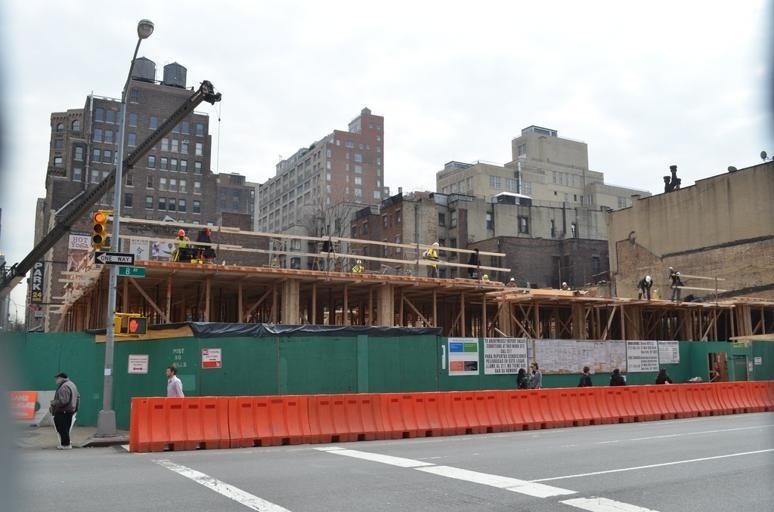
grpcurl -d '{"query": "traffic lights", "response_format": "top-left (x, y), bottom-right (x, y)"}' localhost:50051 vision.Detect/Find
top-left (92, 209), bottom-right (108, 248)
top-left (113, 314), bottom-right (147, 341)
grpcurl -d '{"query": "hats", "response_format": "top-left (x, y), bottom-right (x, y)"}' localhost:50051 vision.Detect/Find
top-left (54, 373), bottom-right (68, 379)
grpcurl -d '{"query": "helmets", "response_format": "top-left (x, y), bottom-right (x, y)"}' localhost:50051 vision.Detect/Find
top-left (562, 281), bottom-right (568, 287)
top-left (646, 276), bottom-right (651, 282)
top-left (432, 242), bottom-right (439, 248)
top-left (356, 259), bottom-right (362, 265)
top-left (676, 271), bottom-right (680, 276)
top-left (483, 274), bottom-right (488, 279)
top-left (176, 229), bottom-right (187, 238)
top-left (510, 277), bottom-right (515, 282)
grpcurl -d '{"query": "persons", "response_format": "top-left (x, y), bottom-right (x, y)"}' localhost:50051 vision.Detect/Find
top-left (669, 271), bottom-right (684, 300)
top-left (610, 368), bottom-right (626, 386)
top-left (637, 276), bottom-right (653, 299)
top-left (481, 275), bottom-right (490, 281)
top-left (467, 248), bottom-right (481, 279)
top-left (518, 370), bottom-right (529, 389)
top-left (529, 363), bottom-right (542, 388)
top-left (196, 228), bottom-right (212, 244)
top-left (50, 374), bottom-right (80, 448)
top-left (352, 260), bottom-right (364, 273)
top-left (578, 366), bottom-right (592, 387)
top-left (656, 368), bottom-right (673, 384)
top-left (166, 367), bottom-right (184, 397)
top-left (560, 281), bottom-right (572, 291)
top-left (175, 229), bottom-right (193, 248)
top-left (684, 294), bottom-right (705, 303)
top-left (427, 242), bottom-right (440, 278)
top-left (506, 278), bottom-right (517, 287)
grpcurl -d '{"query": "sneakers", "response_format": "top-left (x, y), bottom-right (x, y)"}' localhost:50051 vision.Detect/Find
top-left (56, 443), bottom-right (72, 449)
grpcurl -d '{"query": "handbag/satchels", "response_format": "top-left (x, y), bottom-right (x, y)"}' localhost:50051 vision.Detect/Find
top-left (49, 392), bottom-right (81, 416)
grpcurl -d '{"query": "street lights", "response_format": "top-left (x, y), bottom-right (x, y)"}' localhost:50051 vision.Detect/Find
top-left (96, 17), bottom-right (155, 436)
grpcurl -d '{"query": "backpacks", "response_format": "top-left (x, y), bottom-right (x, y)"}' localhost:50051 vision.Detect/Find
top-left (576, 375), bottom-right (588, 387)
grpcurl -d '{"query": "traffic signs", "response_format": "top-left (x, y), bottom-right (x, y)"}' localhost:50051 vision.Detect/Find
top-left (93, 250), bottom-right (134, 266)
